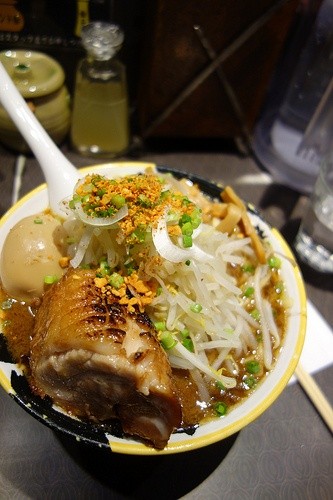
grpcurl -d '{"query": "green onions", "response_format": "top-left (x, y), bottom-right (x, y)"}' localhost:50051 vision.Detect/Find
top-left (34, 171), bottom-right (284, 416)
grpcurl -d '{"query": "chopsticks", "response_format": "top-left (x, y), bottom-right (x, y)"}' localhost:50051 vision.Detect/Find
top-left (293, 362), bottom-right (333, 434)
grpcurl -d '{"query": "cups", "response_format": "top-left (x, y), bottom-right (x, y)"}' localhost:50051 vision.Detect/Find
top-left (294, 157), bottom-right (333, 272)
top-left (250, 0), bottom-right (333, 195)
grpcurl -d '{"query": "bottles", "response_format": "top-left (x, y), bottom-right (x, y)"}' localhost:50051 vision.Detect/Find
top-left (72, 20), bottom-right (130, 158)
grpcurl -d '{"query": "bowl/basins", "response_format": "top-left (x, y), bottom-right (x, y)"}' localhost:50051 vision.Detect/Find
top-left (0, 159), bottom-right (306, 456)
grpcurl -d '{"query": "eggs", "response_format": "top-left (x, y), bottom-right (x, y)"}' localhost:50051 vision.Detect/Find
top-left (0, 212), bottom-right (71, 301)
